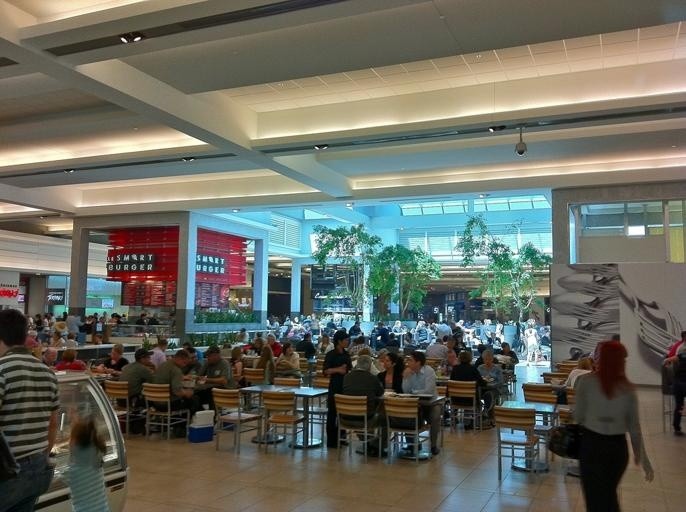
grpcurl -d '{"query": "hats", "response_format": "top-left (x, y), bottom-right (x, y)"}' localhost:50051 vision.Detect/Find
top-left (204, 346), bottom-right (222, 355)
top-left (135, 349), bottom-right (154, 360)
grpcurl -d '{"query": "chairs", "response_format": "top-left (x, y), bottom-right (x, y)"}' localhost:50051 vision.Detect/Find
top-left (105, 351), bottom-right (517, 467)
top-left (494, 360), bottom-right (593, 483)
top-left (660, 355), bottom-right (686, 435)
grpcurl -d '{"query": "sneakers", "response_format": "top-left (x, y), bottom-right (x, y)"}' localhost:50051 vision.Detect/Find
top-left (430, 446), bottom-right (440, 455)
top-left (402, 443), bottom-right (423, 451)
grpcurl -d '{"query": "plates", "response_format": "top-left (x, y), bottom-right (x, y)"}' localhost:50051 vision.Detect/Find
top-left (384, 390), bottom-right (435, 399)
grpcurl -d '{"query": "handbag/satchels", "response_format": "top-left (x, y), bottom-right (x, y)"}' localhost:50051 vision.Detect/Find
top-left (546, 423), bottom-right (590, 460)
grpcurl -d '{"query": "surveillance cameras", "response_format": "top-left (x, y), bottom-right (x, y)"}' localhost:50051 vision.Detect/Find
top-left (515, 144), bottom-right (529, 156)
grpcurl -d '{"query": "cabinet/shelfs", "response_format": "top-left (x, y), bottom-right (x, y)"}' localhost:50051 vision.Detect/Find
top-left (25, 370), bottom-right (129, 511)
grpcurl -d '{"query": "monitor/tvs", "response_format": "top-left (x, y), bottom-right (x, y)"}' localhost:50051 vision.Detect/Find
top-left (17, 294), bottom-right (25, 303)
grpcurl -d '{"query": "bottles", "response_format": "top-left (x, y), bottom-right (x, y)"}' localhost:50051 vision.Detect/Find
top-left (436, 365), bottom-right (443, 379)
